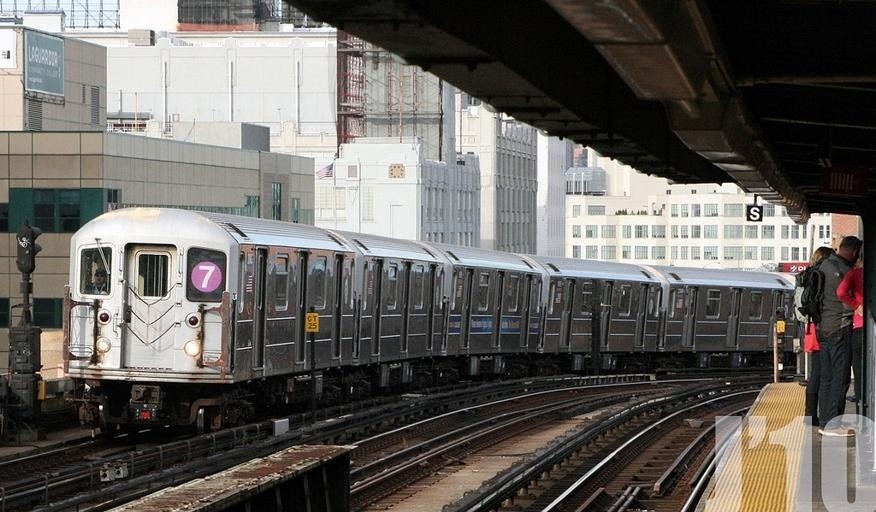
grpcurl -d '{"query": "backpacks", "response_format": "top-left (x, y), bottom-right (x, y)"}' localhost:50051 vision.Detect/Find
top-left (797, 256), bottom-right (830, 325)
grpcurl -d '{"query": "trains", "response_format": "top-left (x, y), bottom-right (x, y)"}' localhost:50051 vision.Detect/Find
top-left (57, 205), bottom-right (798, 444)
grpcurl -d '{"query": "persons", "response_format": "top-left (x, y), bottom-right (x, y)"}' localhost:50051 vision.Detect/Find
top-left (835, 254), bottom-right (865, 423)
top-left (793, 246), bottom-right (837, 427)
top-left (811, 235), bottom-right (863, 438)
top-left (83, 266), bottom-right (107, 295)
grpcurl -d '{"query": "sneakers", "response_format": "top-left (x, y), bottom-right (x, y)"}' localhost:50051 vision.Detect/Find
top-left (818, 426), bottom-right (856, 438)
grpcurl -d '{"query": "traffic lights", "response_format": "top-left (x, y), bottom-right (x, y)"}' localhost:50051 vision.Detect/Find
top-left (15, 221), bottom-right (44, 274)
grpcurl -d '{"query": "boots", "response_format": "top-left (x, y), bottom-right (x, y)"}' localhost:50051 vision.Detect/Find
top-left (804, 388), bottom-right (820, 425)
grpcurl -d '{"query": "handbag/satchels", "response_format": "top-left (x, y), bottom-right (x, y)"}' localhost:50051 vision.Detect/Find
top-left (804, 321), bottom-right (820, 353)
